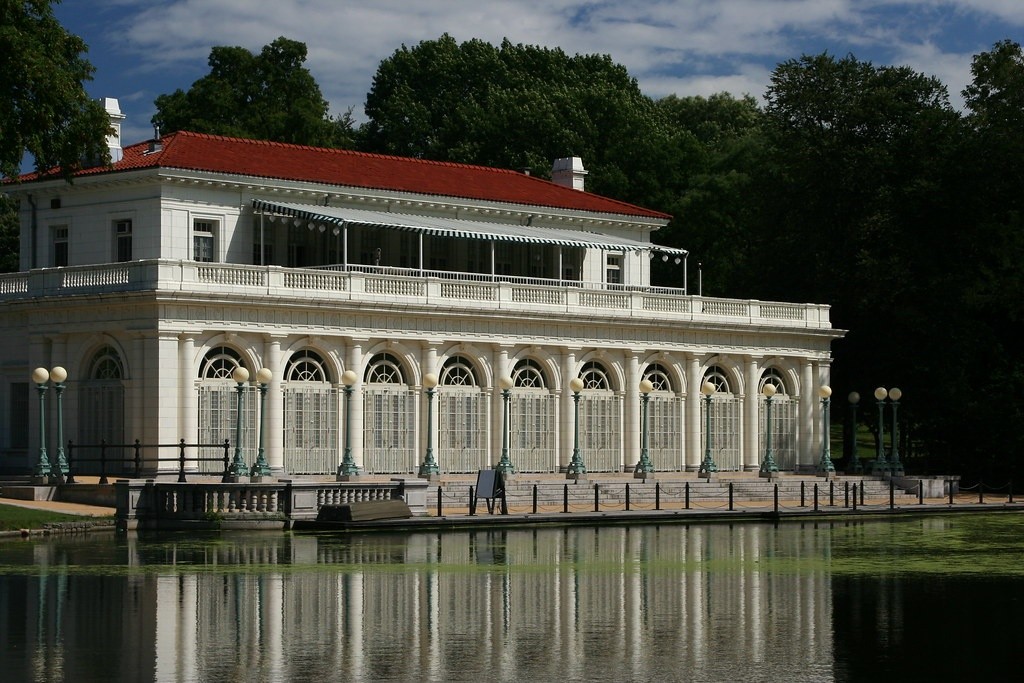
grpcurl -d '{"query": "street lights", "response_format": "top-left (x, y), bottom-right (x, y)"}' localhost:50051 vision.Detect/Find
top-left (815, 385), bottom-right (837, 479)
top-left (758, 384), bottom-right (781, 479)
top-left (221, 366), bottom-right (250, 483)
top-left (695, 382), bottom-right (719, 478)
top-left (32, 368), bottom-right (53, 486)
top-left (334, 370), bottom-right (361, 482)
top-left (869, 388), bottom-right (891, 477)
top-left (249, 368), bottom-right (273, 484)
top-left (634, 379), bottom-right (657, 480)
top-left (495, 376), bottom-right (522, 481)
top-left (568, 379), bottom-right (587, 480)
top-left (48, 367), bottom-right (71, 485)
top-left (842, 391), bottom-right (867, 476)
top-left (887, 388), bottom-right (907, 478)
top-left (417, 374), bottom-right (443, 480)
